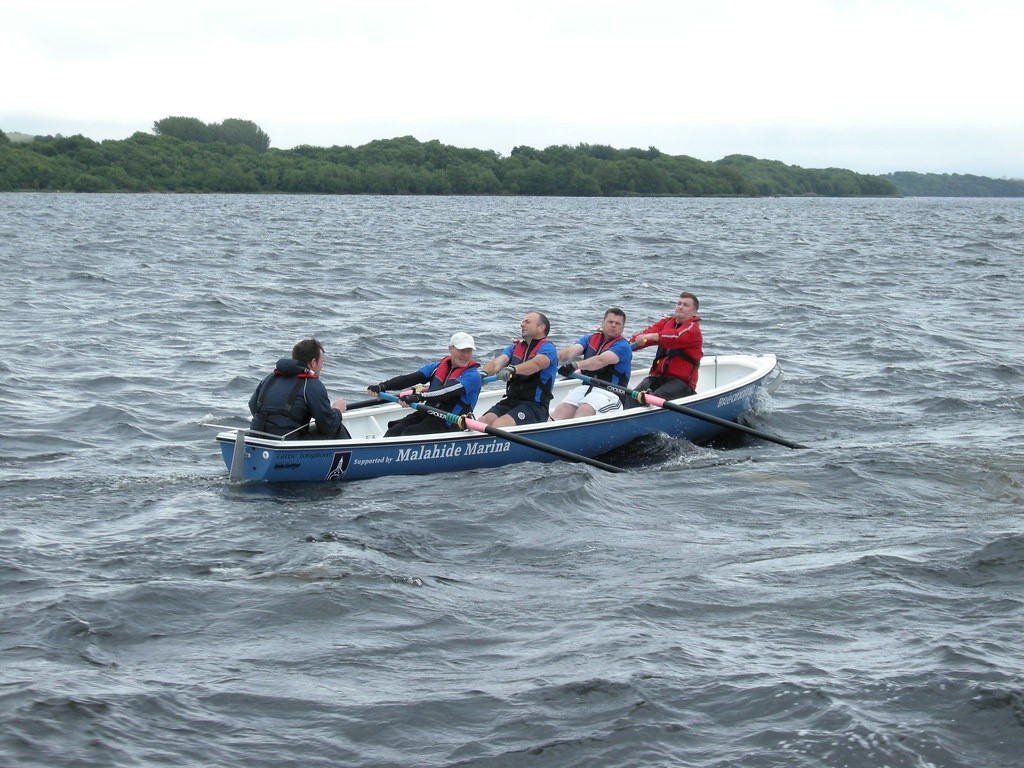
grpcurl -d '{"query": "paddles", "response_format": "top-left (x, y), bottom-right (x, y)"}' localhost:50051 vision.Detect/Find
top-left (484, 371), bottom-right (513, 386)
top-left (380, 391), bottom-right (627, 473)
top-left (564, 373), bottom-right (811, 451)
top-left (345, 390), bottom-right (414, 412)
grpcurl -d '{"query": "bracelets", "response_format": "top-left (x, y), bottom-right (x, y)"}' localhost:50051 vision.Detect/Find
top-left (509, 365), bottom-right (516, 374)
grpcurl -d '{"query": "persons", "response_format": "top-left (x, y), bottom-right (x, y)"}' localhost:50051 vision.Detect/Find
top-left (249, 339), bottom-right (347, 440)
top-left (367, 333), bottom-right (481, 438)
top-left (553, 308), bottom-right (632, 420)
top-left (478, 312), bottom-right (558, 428)
top-left (626, 293), bottom-right (703, 409)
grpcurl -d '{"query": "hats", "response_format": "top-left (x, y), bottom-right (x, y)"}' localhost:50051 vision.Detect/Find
top-left (449, 332), bottom-right (477, 351)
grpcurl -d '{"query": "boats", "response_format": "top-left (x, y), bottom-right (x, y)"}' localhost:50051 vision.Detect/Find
top-left (215, 353), bottom-right (784, 482)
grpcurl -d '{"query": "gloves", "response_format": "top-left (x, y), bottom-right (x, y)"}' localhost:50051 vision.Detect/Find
top-left (498, 363), bottom-right (516, 382)
top-left (558, 362), bottom-right (578, 378)
